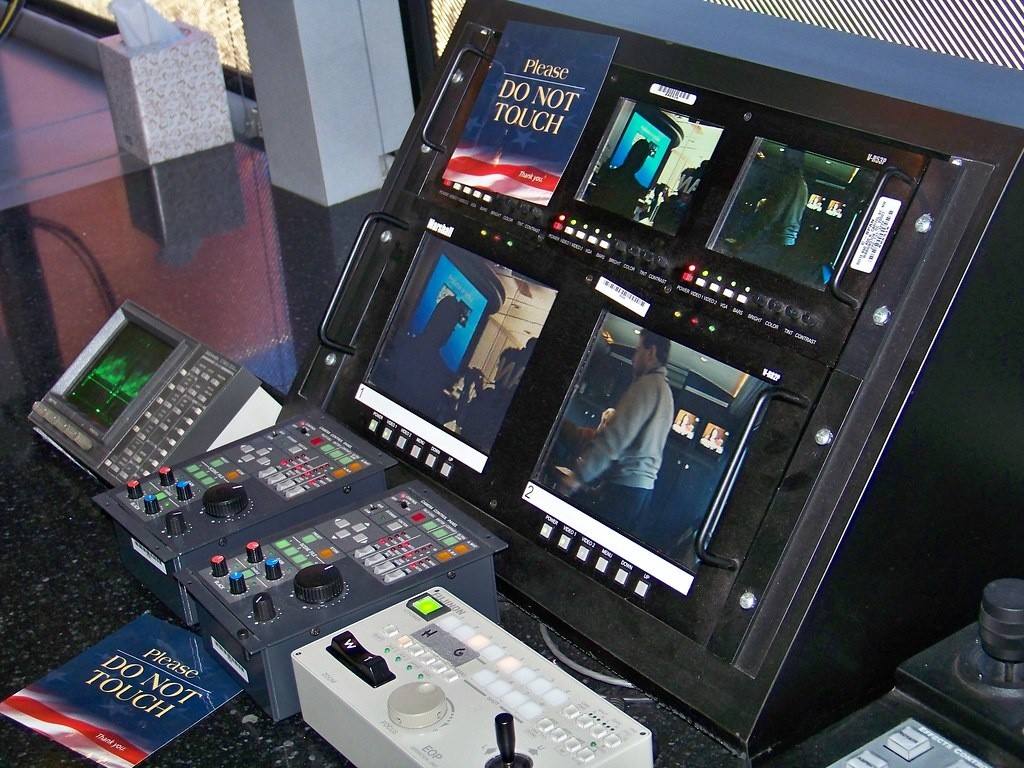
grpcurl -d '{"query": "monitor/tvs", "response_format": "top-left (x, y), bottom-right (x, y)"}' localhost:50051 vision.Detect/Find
top-left (323, 60), bottom-right (936, 660)
top-left (66, 321), bottom-right (180, 430)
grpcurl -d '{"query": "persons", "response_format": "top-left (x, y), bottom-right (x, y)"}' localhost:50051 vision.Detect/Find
top-left (388, 296), bottom-right (466, 426)
top-left (593, 139), bottom-right (650, 221)
top-left (557, 328), bottom-right (675, 530)
top-left (733, 146), bottom-right (809, 270)
top-left (456, 347), bottom-right (533, 457)
top-left (652, 167), bottom-right (699, 237)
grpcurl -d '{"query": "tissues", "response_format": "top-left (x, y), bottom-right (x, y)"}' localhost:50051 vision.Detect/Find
top-left (96, 0), bottom-right (237, 164)
top-left (118, 145), bottom-right (250, 269)
